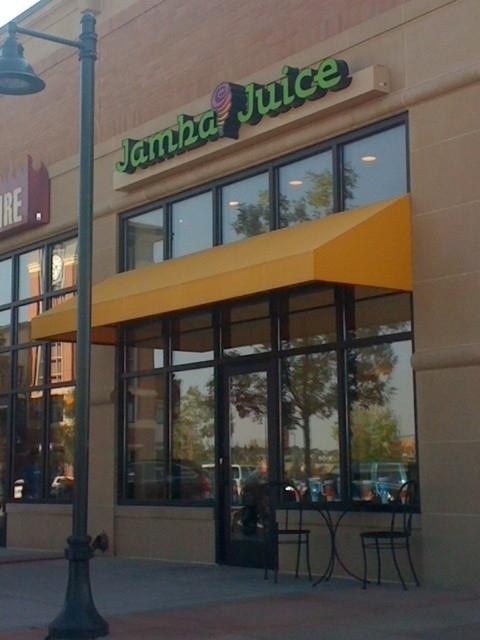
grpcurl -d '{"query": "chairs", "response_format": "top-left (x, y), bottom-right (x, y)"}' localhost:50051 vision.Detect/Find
top-left (260, 481), bottom-right (313, 586)
top-left (359, 478), bottom-right (421, 592)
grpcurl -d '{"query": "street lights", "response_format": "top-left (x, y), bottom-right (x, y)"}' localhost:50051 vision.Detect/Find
top-left (0, 8), bottom-right (116, 635)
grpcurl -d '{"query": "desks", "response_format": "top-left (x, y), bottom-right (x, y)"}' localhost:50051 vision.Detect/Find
top-left (307, 500), bottom-right (372, 586)
